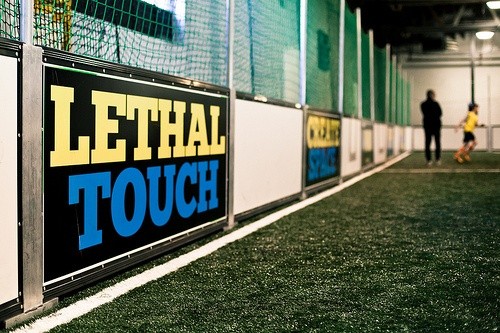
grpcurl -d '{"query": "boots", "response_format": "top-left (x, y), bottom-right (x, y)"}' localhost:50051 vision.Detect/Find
top-left (453, 147), bottom-right (472, 164)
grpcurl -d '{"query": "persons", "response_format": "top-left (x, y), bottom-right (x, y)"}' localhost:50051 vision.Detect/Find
top-left (455, 102), bottom-right (485, 163)
top-left (419, 89), bottom-right (444, 167)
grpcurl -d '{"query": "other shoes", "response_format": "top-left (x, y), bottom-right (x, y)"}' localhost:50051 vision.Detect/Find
top-left (425, 160), bottom-right (441, 166)
